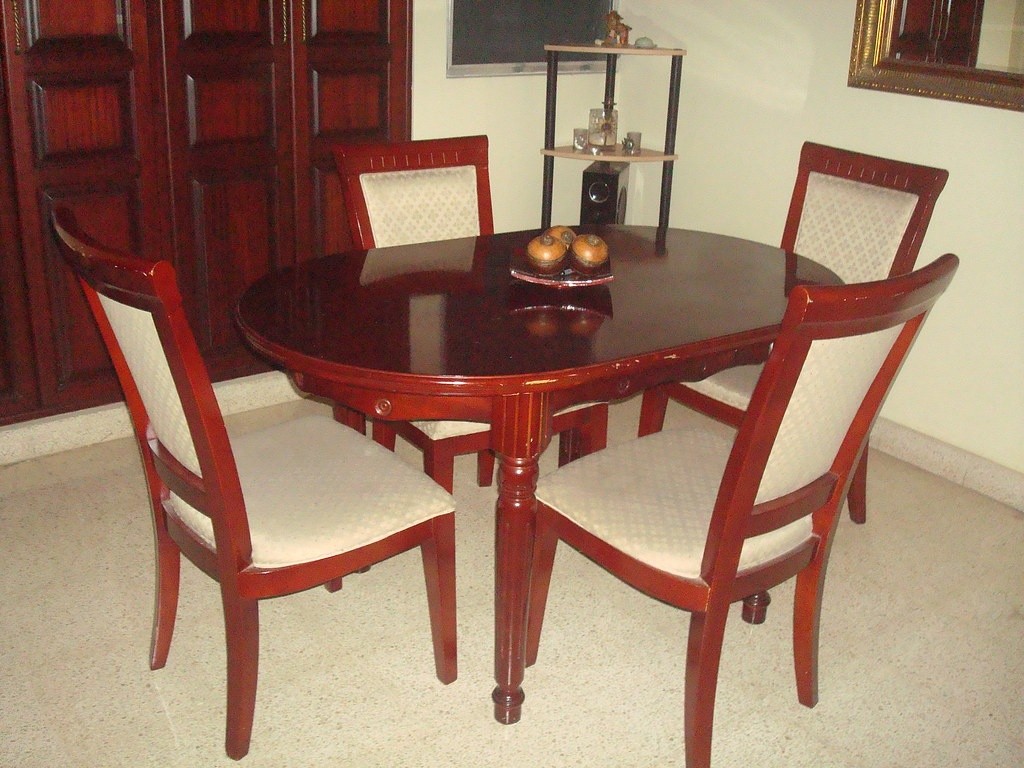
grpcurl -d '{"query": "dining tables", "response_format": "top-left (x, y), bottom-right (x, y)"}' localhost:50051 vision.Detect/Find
top-left (233, 222), bottom-right (846, 726)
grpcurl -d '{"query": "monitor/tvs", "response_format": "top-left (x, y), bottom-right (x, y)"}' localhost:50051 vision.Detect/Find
top-left (446, 0), bottom-right (613, 79)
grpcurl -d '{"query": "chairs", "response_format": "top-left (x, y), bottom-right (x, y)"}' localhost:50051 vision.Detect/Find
top-left (521, 253), bottom-right (959, 768)
top-left (636, 139), bottom-right (950, 625)
top-left (50, 200), bottom-right (460, 761)
top-left (335, 135), bottom-right (607, 575)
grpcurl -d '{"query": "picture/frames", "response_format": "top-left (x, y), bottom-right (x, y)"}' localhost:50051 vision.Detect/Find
top-left (847, 0), bottom-right (1024, 115)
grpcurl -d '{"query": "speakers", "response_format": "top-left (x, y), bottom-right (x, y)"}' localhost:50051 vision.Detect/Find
top-left (580, 161), bottom-right (630, 225)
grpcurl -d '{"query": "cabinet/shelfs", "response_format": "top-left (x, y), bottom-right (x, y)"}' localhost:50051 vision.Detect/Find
top-left (541, 43), bottom-right (685, 226)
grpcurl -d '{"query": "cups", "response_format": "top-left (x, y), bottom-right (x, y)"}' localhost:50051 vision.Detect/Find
top-left (590, 109), bottom-right (617, 150)
top-left (573, 130), bottom-right (588, 151)
top-left (627, 133), bottom-right (641, 151)
